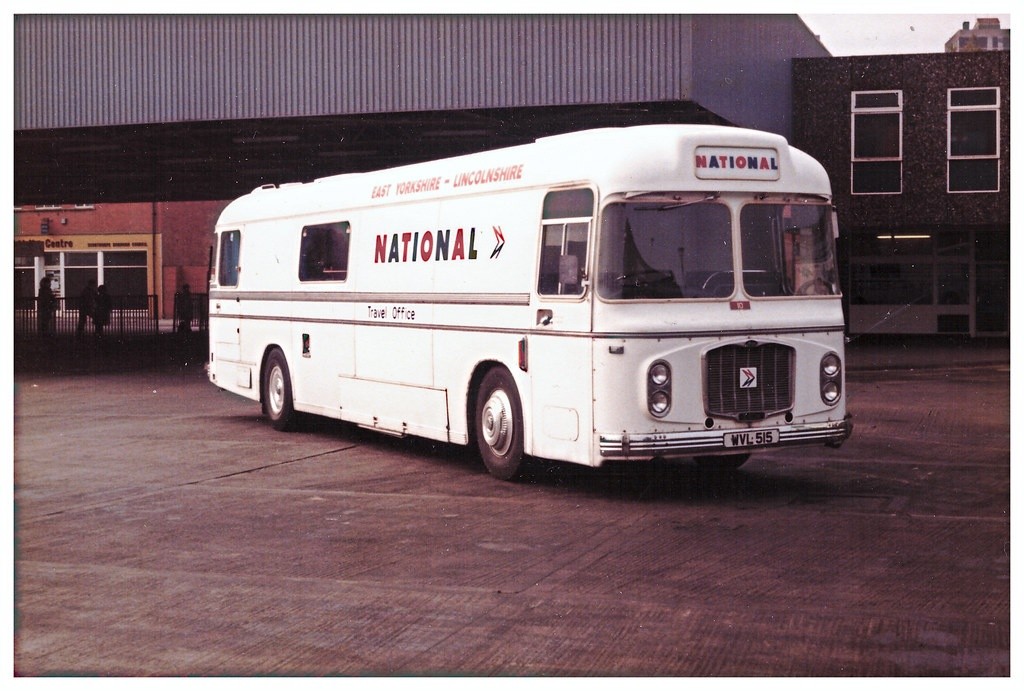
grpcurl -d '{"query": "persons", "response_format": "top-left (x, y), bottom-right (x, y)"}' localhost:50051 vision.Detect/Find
top-left (176, 284), bottom-right (193, 332)
top-left (37, 277), bottom-right (113, 338)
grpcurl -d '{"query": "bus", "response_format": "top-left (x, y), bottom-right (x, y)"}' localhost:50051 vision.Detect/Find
top-left (205, 123), bottom-right (856, 479)
